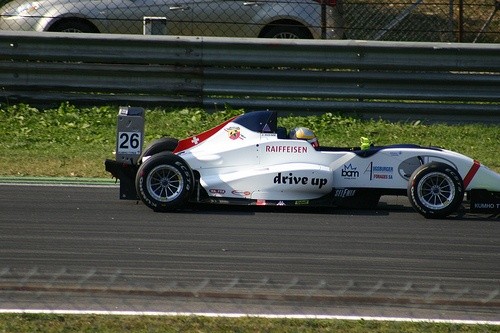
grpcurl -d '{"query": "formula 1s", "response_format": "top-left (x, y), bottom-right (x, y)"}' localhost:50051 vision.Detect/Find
top-left (105, 109), bottom-right (500, 220)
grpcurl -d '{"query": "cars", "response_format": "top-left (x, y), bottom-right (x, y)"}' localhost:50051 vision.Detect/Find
top-left (0, 0), bottom-right (343, 71)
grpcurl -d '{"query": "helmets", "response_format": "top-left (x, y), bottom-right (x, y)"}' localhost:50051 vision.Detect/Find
top-left (289, 126), bottom-right (319, 148)
